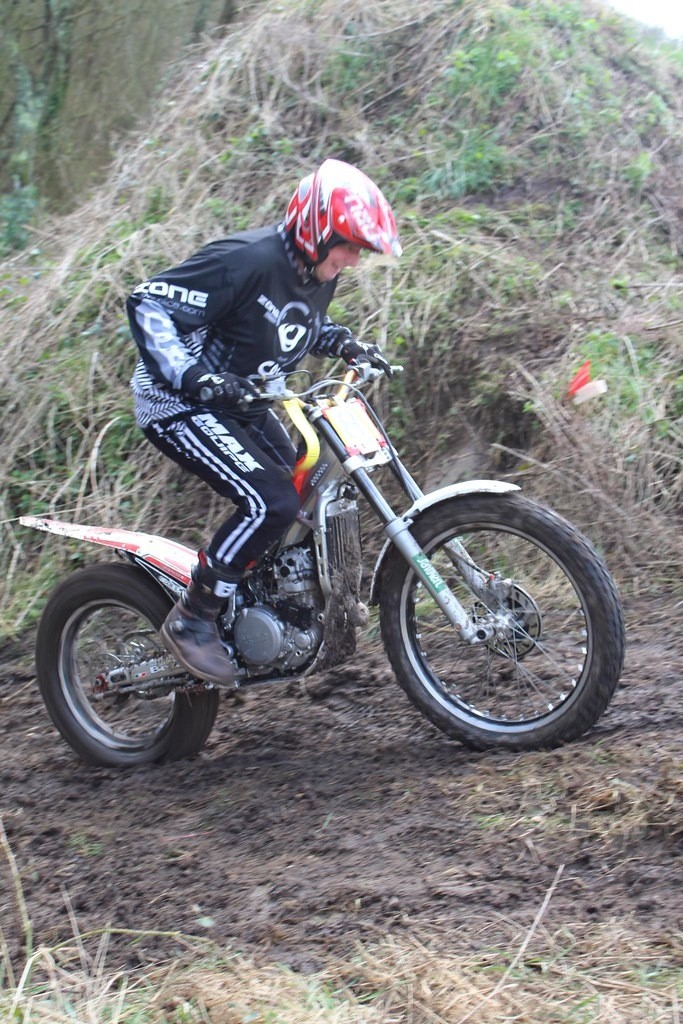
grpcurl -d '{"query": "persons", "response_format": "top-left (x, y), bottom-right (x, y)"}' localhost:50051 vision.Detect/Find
top-left (126, 156), bottom-right (403, 691)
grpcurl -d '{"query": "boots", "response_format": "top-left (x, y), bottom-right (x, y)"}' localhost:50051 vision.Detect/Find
top-left (159, 547), bottom-right (256, 686)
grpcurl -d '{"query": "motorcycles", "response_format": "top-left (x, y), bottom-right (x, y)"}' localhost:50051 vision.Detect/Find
top-left (16, 360), bottom-right (630, 769)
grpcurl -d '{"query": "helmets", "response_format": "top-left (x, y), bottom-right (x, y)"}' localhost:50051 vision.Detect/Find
top-left (285, 159), bottom-right (403, 268)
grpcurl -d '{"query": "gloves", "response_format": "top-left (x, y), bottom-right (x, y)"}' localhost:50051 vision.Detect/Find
top-left (184, 364), bottom-right (260, 407)
top-left (332, 332), bottom-right (393, 383)
